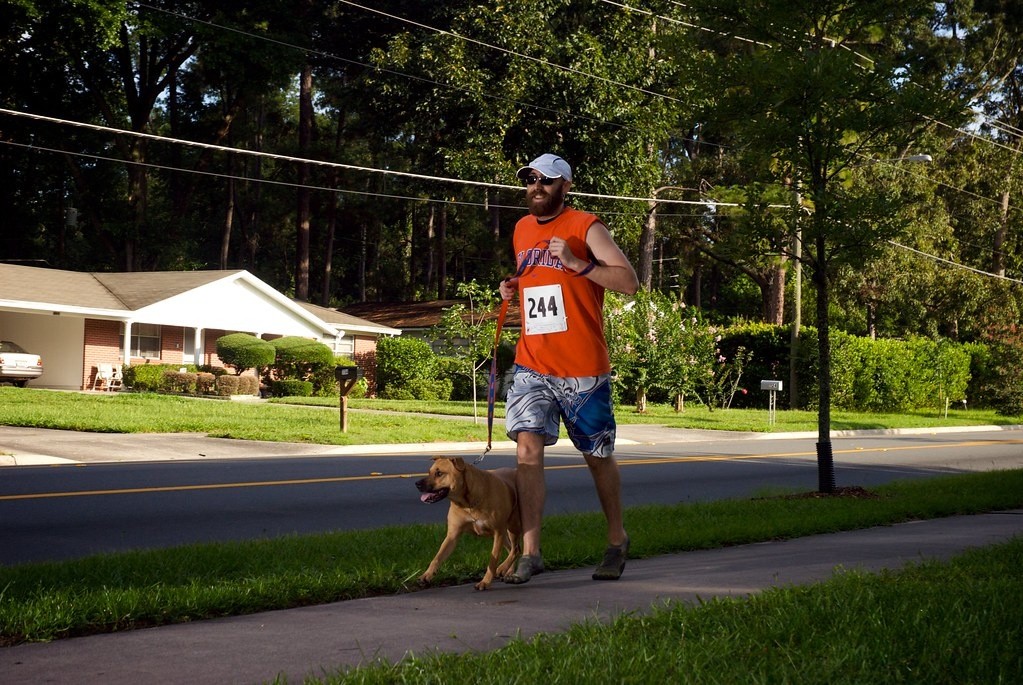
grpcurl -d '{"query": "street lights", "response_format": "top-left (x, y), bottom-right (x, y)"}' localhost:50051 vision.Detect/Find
top-left (787, 152), bottom-right (935, 410)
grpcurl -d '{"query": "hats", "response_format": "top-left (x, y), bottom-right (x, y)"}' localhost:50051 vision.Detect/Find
top-left (516, 153), bottom-right (572, 183)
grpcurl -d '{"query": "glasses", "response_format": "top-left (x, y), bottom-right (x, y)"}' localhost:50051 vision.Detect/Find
top-left (525, 176), bottom-right (563, 185)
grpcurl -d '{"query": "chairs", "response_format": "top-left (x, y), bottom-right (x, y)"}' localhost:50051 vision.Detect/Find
top-left (92, 363), bottom-right (133, 393)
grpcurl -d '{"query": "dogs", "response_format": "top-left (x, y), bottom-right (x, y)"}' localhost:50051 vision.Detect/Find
top-left (416, 456), bottom-right (521, 591)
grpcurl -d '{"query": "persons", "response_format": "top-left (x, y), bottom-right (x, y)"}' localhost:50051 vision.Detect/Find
top-left (499, 154), bottom-right (640, 584)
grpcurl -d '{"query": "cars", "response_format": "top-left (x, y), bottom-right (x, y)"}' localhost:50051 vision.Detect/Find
top-left (0, 339), bottom-right (43, 388)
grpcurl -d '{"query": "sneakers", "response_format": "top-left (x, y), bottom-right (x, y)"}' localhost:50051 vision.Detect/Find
top-left (592, 534), bottom-right (630, 580)
top-left (505, 548), bottom-right (545, 584)
